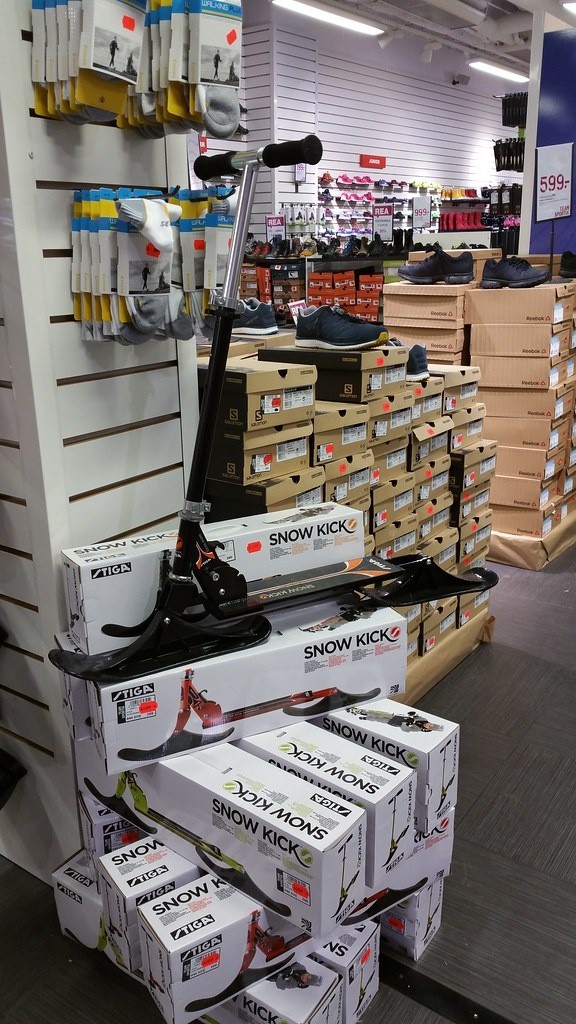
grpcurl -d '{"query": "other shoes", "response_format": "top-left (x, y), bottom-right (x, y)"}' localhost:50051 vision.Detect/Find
top-left (414, 240), bottom-right (488, 250)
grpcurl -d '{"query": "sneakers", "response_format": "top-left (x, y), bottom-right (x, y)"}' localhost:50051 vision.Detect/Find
top-left (319, 188), bottom-right (443, 207)
top-left (318, 170), bottom-right (443, 190)
top-left (295, 305), bottom-right (389, 350)
top-left (397, 248), bottom-right (475, 284)
top-left (325, 208), bottom-right (412, 221)
top-left (479, 256), bottom-right (547, 288)
top-left (389, 337), bottom-right (430, 382)
top-left (205, 296), bottom-right (278, 334)
top-left (558, 250), bottom-right (576, 277)
top-left (321, 223), bottom-right (373, 237)
top-left (244, 233), bottom-right (386, 260)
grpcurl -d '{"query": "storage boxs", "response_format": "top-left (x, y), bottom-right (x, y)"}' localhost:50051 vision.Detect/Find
top-left (53, 248), bottom-right (576, 1024)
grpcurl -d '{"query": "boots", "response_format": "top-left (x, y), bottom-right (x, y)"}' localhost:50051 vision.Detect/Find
top-left (440, 188), bottom-right (481, 201)
top-left (387, 229), bottom-right (414, 256)
top-left (439, 211), bottom-right (487, 230)
